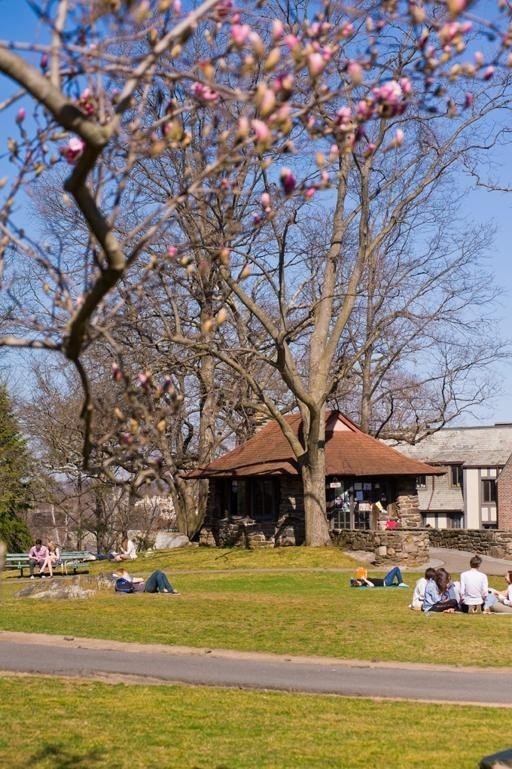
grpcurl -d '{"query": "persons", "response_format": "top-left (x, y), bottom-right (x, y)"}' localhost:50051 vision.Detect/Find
top-left (28, 538), bottom-right (60, 580)
top-left (116, 537), bottom-right (137, 561)
top-left (411, 555), bottom-right (512, 613)
top-left (350, 567), bottom-right (409, 587)
top-left (114, 567), bottom-right (181, 595)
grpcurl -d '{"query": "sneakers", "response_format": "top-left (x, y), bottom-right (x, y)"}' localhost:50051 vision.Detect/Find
top-left (468, 604), bottom-right (482, 614)
top-left (398, 582), bottom-right (408, 588)
top-left (30, 575), bottom-right (46, 580)
top-left (161, 590), bottom-right (182, 596)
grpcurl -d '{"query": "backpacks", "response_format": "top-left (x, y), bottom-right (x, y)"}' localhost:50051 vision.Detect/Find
top-left (114, 578), bottom-right (134, 592)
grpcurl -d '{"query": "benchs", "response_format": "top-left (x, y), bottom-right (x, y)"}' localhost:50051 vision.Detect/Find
top-left (4, 551), bottom-right (89, 577)
